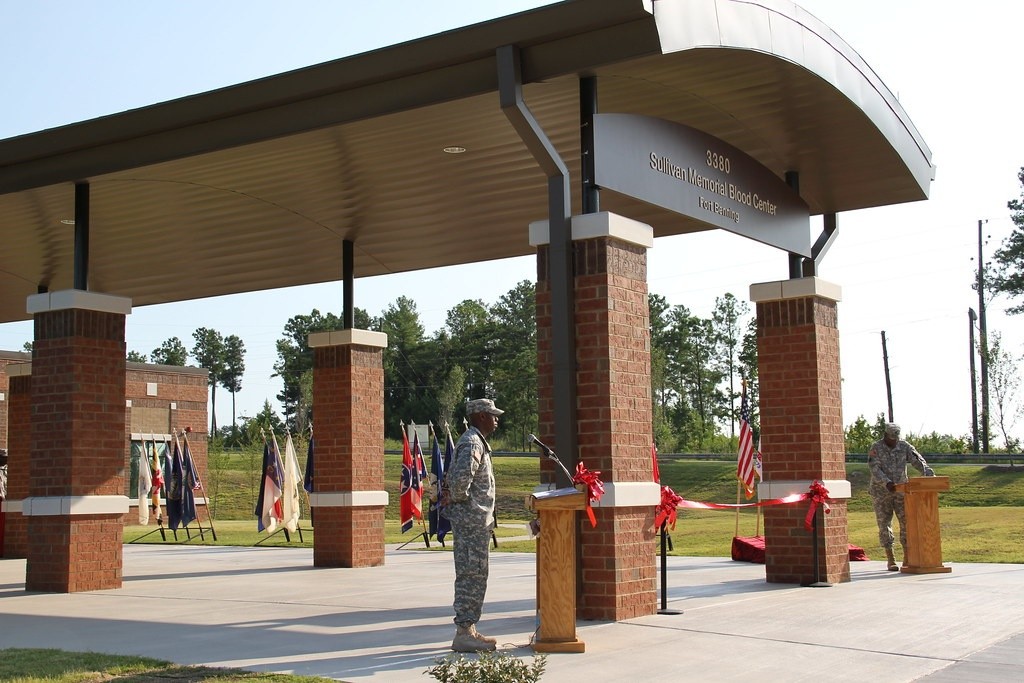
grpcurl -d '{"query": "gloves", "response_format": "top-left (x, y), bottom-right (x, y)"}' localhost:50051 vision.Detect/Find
top-left (886, 481), bottom-right (897, 493)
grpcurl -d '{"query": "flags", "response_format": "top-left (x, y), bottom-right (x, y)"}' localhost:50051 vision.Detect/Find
top-left (138, 437), bottom-right (199, 533)
top-left (400, 429), bottom-right (455, 543)
top-left (737, 387), bottom-right (762, 501)
top-left (255, 435), bottom-right (314, 533)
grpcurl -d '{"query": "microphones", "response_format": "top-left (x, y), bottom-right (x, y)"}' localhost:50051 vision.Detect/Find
top-left (911, 450), bottom-right (919, 459)
top-left (527, 434), bottom-right (554, 455)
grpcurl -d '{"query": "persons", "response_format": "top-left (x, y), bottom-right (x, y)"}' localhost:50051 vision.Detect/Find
top-left (868, 423), bottom-right (935, 571)
top-left (440, 399), bottom-right (505, 653)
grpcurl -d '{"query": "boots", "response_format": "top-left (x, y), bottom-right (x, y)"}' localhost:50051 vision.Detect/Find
top-left (473, 622), bottom-right (496, 644)
top-left (886, 548), bottom-right (898, 571)
top-left (451, 624), bottom-right (488, 652)
top-left (902, 545), bottom-right (907, 566)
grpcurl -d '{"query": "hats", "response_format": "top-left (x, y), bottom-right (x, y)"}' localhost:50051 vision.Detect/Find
top-left (885, 423), bottom-right (901, 439)
top-left (466, 398), bottom-right (505, 416)
